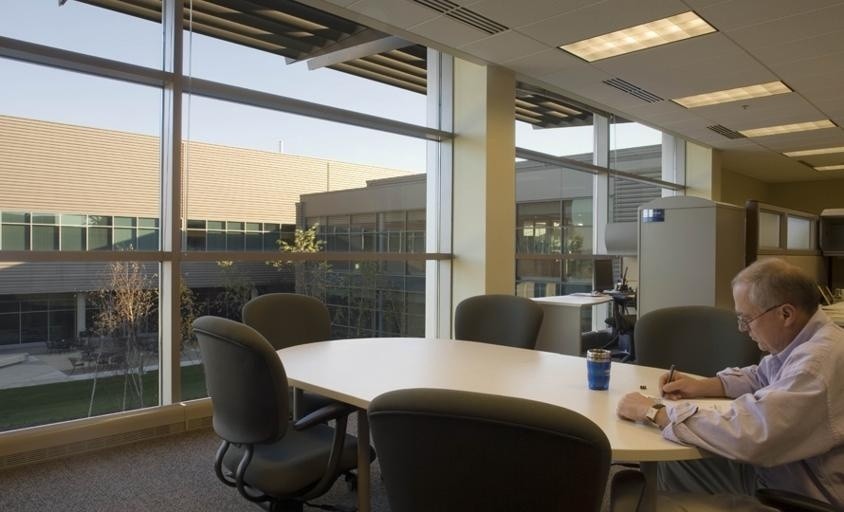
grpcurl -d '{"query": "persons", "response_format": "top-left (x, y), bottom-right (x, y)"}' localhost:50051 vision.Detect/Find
top-left (617, 258), bottom-right (844, 512)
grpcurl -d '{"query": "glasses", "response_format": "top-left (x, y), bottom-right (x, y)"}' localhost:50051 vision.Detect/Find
top-left (737, 302), bottom-right (796, 330)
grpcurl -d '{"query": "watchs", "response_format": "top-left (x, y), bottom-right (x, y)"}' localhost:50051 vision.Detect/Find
top-left (644, 402), bottom-right (665, 423)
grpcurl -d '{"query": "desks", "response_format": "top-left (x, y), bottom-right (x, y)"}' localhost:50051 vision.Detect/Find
top-left (524, 293), bottom-right (633, 358)
top-left (277, 338), bottom-right (738, 510)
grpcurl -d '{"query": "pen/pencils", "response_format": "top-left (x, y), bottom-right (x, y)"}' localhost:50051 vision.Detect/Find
top-left (662, 364), bottom-right (676, 398)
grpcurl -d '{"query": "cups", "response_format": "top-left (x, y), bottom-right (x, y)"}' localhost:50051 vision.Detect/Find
top-left (587, 349), bottom-right (611, 391)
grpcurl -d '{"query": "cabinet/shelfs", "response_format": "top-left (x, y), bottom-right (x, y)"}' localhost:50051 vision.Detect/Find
top-left (636, 196), bottom-right (745, 323)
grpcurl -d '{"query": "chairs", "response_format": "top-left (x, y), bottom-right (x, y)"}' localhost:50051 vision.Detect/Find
top-left (190, 316), bottom-right (375, 506)
top-left (366, 387), bottom-right (647, 511)
top-left (456, 294), bottom-right (543, 348)
top-left (242, 291), bottom-right (357, 493)
top-left (605, 286), bottom-right (637, 364)
top-left (755, 488), bottom-right (844, 512)
top-left (634, 307), bottom-right (759, 379)
